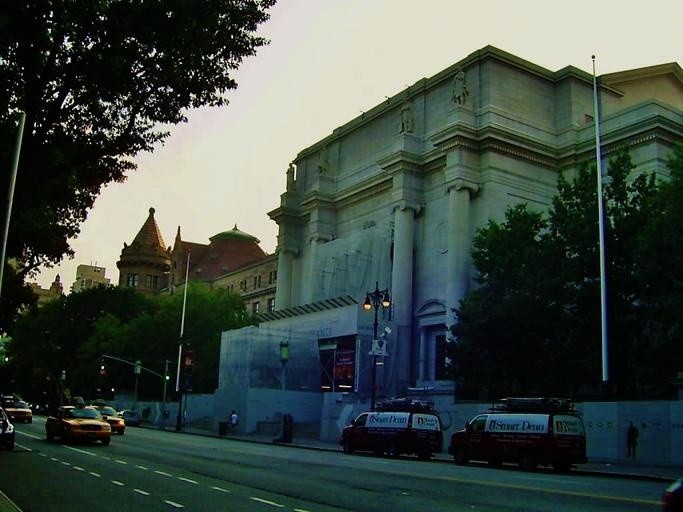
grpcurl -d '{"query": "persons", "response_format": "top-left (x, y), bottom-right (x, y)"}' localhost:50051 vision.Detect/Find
top-left (229, 410), bottom-right (238, 435)
top-left (452, 65), bottom-right (468, 104)
top-left (400, 99), bottom-right (413, 133)
top-left (286, 163), bottom-right (294, 186)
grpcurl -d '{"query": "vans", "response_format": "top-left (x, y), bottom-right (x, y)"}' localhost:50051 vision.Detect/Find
top-left (337, 396), bottom-right (443, 460)
top-left (448, 396), bottom-right (586, 472)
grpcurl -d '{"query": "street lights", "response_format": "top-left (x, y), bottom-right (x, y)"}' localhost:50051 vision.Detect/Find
top-left (174, 246), bottom-right (192, 392)
top-left (590, 54), bottom-right (615, 385)
top-left (361, 279), bottom-right (391, 411)
top-left (0, 107), bottom-right (27, 290)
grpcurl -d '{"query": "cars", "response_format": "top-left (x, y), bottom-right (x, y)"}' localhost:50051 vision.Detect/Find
top-left (117, 410), bottom-right (140, 425)
top-left (4, 400), bottom-right (32, 423)
top-left (44, 396), bottom-right (110, 444)
top-left (88, 398), bottom-right (125, 436)
top-left (0, 405), bottom-right (15, 449)
top-left (662, 476), bottom-right (683, 511)
top-left (26, 401), bottom-right (50, 413)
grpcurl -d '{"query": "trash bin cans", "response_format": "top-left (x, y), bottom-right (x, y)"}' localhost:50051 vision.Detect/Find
top-left (219, 422), bottom-right (227, 436)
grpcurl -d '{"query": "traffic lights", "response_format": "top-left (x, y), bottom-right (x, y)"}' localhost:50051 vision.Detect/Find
top-left (3, 356), bottom-right (8, 362)
top-left (165, 375), bottom-right (169, 380)
top-left (100, 364), bottom-right (105, 375)
